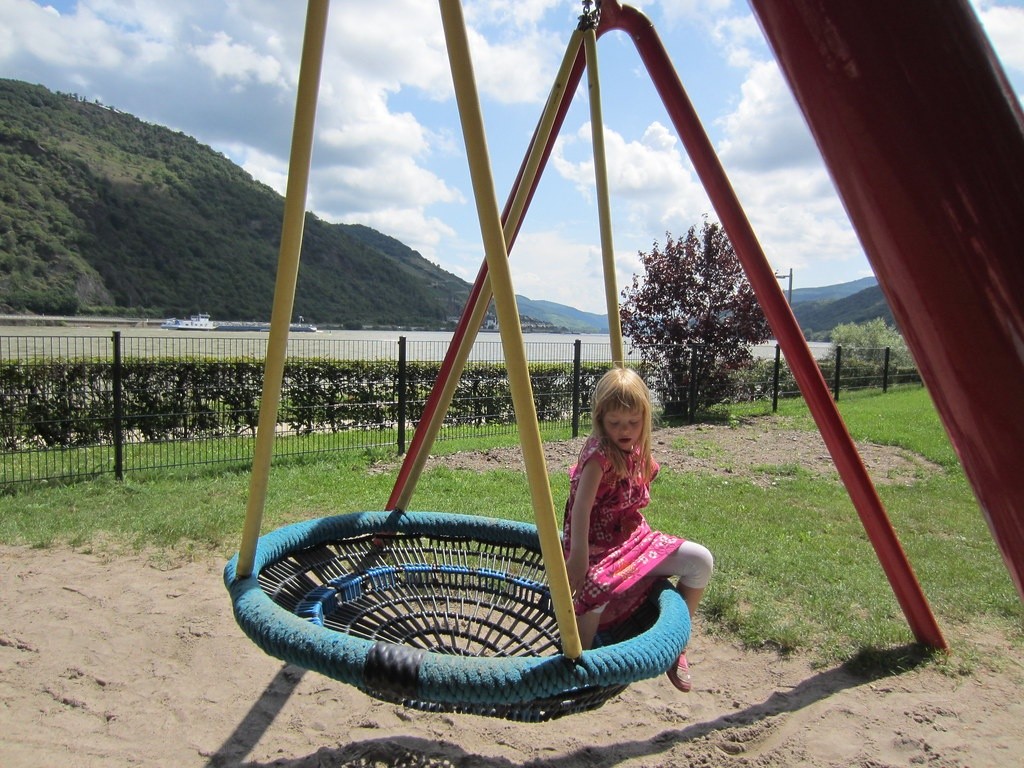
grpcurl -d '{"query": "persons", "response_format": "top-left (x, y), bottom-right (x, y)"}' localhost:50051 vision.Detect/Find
top-left (562, 368), bottom-right (715, 694)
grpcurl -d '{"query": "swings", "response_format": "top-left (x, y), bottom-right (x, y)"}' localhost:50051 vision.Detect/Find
top-left (224, 1), bottom-right (692, 721)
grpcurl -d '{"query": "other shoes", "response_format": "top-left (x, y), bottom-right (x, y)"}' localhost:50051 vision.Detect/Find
top-left (666, 648), bottom-right (692, 692)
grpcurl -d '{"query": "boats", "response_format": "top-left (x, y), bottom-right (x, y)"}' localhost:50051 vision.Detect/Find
top-left (161, 312), bottom-right (220, 331)
top-left (259, 316), bottom-right (319, 333)
